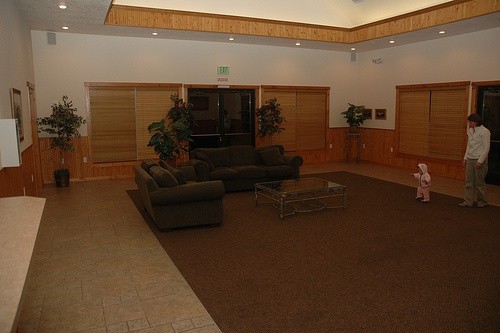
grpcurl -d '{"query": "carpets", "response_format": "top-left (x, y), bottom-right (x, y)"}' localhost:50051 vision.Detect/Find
top-left (126, 170), bottom-right (500, 333)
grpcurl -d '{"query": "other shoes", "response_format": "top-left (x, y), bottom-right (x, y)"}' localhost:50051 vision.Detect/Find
top-left (458, 199), bottom-right (474, 208)
top-left (415, 196), bottom-right (421, 200)
top-left (476, 200), bottom-right (489, 207)
top-left (421, 199), bottom-right (428, 203)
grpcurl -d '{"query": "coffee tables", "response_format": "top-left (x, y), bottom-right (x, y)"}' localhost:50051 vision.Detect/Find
top-left (255, 177), bottom-right (347, 219)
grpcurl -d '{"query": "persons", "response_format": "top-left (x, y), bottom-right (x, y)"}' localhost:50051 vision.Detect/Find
top-left (458, 112), bottom-right (491, 207)
top-left (411, 164), bottom-right (432, 203)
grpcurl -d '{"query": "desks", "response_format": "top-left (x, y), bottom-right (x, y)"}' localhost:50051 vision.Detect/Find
top-left (345, 132), bottom-right (360, 161)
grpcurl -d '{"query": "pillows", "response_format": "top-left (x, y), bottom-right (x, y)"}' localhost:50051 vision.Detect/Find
top-left (196, 152), bottom-right (215, 171)
top-left (261, 148), bottom-right (287, 165)
top-left (160, 160), bottom-right (187, 184)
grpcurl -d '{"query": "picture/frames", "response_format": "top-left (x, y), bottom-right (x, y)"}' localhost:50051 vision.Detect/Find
top-left (375, 109), bottom-right (386, 120)
top-left (10, 88), bottom-right (24, 142)
top-left (364, 109), bottom-right (372, 119)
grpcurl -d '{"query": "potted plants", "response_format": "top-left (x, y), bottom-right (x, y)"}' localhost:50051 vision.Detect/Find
top-left (36, 96), bottom-right (88, 188)
top-left (341, 103), bottom-right (368, 134)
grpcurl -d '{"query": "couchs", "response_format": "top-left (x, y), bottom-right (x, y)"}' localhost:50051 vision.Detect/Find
top-left (184, 145), bottom-right (303, 193)
top-left (133, 159), bottom-right (225, 231)
